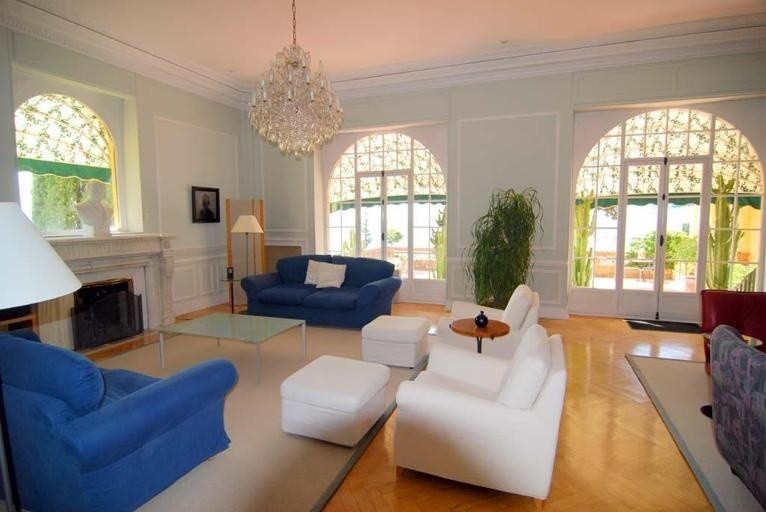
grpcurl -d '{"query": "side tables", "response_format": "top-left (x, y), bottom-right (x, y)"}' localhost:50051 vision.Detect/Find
top-left (220, 277), bottom-right (241, 313)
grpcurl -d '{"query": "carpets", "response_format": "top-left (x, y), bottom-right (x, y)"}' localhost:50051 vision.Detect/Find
top-left (624, 353), bottom-right (766, 512)
top-left (624, 318), bottom-right (704, 334)
top-left (90, 313), bottom-right (429, 511)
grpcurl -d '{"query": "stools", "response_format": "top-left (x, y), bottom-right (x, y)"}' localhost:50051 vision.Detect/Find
top-left (279, 354), bottom-right (389, 448)
top-left (361, 314), bottom-right (432, 369)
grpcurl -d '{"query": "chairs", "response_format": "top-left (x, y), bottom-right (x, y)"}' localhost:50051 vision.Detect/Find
top-left (393, 284), bottom-right (568, 512)
top-left (0, 329), bottom-right (239, 511)
top-left (709, 324), bottom-right (766, 508)
top-left (700, 289), bottom-right (765, 376)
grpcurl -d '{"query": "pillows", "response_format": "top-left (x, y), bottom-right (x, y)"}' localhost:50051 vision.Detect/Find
top-left (303, 259), bottom-right (348, 289)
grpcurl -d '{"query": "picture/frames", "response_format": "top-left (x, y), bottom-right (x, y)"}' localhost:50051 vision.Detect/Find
top-left (189, 185), bottom-right (221, 223)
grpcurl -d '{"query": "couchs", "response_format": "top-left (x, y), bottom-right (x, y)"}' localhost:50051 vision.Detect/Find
top-left (240, 254), bottom-right (402, 329)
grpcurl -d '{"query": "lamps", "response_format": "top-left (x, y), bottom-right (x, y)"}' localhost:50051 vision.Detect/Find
top-left (0, 201), bottom-right (82, 309)
top-left (248, 0), bottom-right (342, 158)
top-left (230, 215), bottom-right (264, 280)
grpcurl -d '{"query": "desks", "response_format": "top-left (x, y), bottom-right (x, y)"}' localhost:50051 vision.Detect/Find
top-left (701, 332), bottom-right (763, 418)
